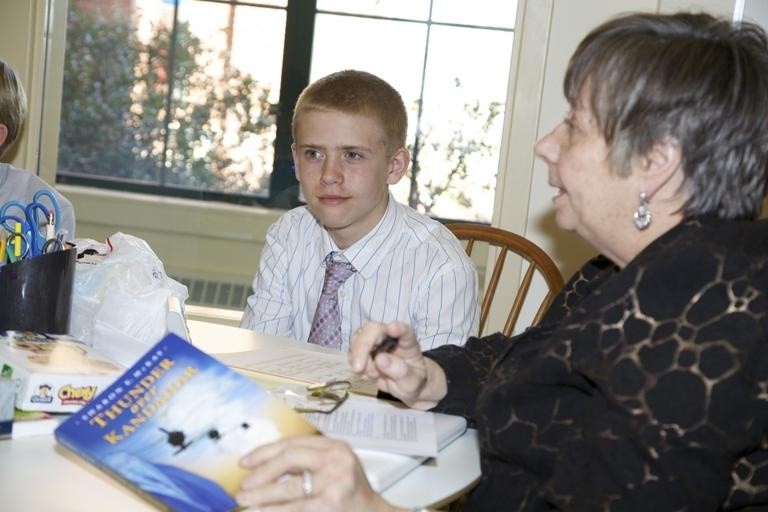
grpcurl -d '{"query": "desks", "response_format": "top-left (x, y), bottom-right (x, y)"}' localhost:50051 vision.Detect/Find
top-left (2, 318), bottom-right (481, 511)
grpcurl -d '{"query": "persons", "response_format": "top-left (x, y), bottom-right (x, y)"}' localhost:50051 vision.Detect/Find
top-left (235, 71), bottom-right (482, 350)
top-left (235, 10), bottom-right (768, 512)
top-left (1, 57), bottom-right (77, 252)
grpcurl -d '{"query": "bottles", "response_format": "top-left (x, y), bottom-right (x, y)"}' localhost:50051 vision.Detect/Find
top-left (1, 364), bottom-right (14, 433)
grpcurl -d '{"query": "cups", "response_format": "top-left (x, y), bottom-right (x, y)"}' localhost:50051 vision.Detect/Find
top-left (1, 248), bottom-right (76, 335)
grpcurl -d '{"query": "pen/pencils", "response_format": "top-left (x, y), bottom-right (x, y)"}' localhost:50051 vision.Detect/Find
top-left (0, 222), bottom-right (21, 267)
top-left (46, 209), bottom-right (76, 253)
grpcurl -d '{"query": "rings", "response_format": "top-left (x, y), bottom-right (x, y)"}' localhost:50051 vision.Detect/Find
top-left (302, 467), bottom-right (313, 495)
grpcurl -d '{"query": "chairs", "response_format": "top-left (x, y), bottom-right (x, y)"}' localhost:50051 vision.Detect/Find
top-left (441, 221), bottom-right (568, 341)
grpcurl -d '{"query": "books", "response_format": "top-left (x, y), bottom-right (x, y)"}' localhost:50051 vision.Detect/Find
top-left (50, 332), bottom-right (328, 512)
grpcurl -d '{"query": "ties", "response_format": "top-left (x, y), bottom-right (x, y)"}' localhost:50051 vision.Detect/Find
top-left (307, 251), bottom-right (358, 351)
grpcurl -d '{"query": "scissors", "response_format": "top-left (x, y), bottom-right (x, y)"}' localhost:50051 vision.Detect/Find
top-left (0, 189), bottom-right (63, 263)
